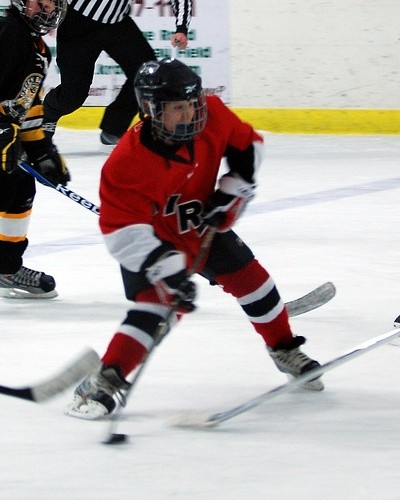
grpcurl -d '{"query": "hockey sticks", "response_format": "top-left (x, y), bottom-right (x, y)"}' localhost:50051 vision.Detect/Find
top-left (0, 347), bottom-right (100, 403)
top-left (17, 161), bottom-right (336, 318)
top-left (98, 217), bottom-right (223, 446)
top-left (169, 326), bottom-right (400, 435)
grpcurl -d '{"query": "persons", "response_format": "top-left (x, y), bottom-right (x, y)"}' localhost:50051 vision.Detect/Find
top-left (0, 0), bottom-right (71, 294)
top-left (42, 0), bottom-right (201, 151)
top-left (72, 57), bottom-right (323, 415)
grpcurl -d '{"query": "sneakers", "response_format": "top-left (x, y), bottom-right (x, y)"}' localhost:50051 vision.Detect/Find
top-left (42, 121), bottom-right (57, 137)
top-left (101, 130), bottom-right (120, 145)
top-left (266, 335), bottom-right (324, 391)
top-left (0, 266), bottom-right (58, 299)
top-left (71, 365), bottom-right (133, 419)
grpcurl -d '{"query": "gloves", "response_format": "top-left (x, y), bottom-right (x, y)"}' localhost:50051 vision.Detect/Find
top-left (145, 250), bottom-right (195, 313)
top-left (23, 138), bottom-right (70, 187)
top-left (199, 170), bottom-right (257, 233)
top-left (0, 123), bottom-right (36, 177)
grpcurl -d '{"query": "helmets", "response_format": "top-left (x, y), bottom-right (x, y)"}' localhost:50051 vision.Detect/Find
top-left (133, 57), bottom-right (207, 147)
top-left (11, 0), bottom-right (68, 35)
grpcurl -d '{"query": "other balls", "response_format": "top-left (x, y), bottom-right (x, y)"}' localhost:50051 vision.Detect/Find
top-left (111, 434), bottom-right (125, 441)
